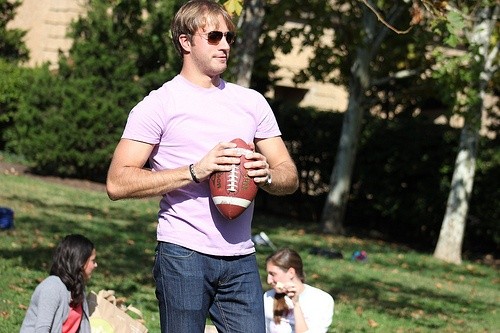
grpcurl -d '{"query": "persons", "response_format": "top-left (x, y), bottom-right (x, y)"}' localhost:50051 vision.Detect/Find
top-left (18, 234), bottom-right (128, 333)
top-left (102, 0), bottom-right (300, 333)
top-left (257, 246), bottom-right (334, 333)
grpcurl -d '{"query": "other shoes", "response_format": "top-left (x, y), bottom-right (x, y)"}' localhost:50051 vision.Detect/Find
top-left (254, 232), bottom-right (277, 251)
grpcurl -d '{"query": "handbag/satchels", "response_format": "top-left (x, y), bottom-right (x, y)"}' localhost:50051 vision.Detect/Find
top-left (85, 289), bottom-right (149, 333)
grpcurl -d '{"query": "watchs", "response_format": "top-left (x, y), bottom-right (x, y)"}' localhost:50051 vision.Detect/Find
top-left (260, 172), bottom-right (273, 188)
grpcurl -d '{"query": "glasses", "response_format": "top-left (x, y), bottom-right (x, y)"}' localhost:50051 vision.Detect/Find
top-left (190, 31), bottom-right (237, 47)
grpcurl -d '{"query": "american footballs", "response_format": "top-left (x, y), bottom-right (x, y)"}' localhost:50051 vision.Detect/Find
top-left (208, 136), bottom-right (260, 223)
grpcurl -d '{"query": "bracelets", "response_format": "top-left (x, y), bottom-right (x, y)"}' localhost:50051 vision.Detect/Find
top-left (189, 163), bottom-right (201, 184)
